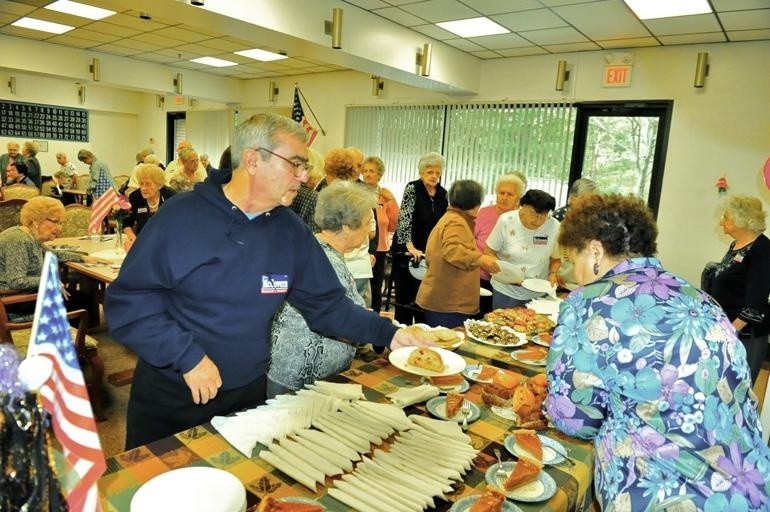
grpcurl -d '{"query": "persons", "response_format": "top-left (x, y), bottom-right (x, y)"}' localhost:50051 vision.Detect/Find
top-left (473, 174), bottom-right (525, 312)
top-left (540, 193), bottom-right (770, 511)
top-left (103, 113), bottom-right (439, 451)
top-left (164, 140), bottom-right (192, 187)
top-left (43, 169), bottom-right (69, 205)
top-left (4, 162), bottom-right (33, 185)
top-left (1, 196), bottom-right (98, 348)
top-left (23, 142), bottom-right (41, 190)
top-left (116, 164), bottom-right (178, 254)
top-left (323, 149), bottom-right (355, 184)
top-left (266, 182), bottom-right (376, 398)
top-left (715, 176), bottom-right (729, 192)
top-left (483, 191), bottom-right (562, 311)
top-left (167, 148), bottom-right (206, 192)
top-left (201, 155), bottom-right (214, 174)
top-left (289, 148), bottom-right (324, 233)
top-left (0, 144), bottom-right (23, 185)
top-left (79, 150), bottom-right (116, 197)
top-left (392, 153), bottom-right (448, 326)
top-left (415, 180), bottom-right (500, 330)
top-left (348, 147), bottom-right (363, 181)
top-left (125, 148), bottom-right (154, 197)
top-left (143, 154), bottom-right (161, 166)
top-left (361, 157), bottom-right (401, 314)
top-left (551, 176), bottom-right (600, 223)
top-left (711, 195), bottom-right (770, 386)
top-left (57, 154), bottom-right (78, 190)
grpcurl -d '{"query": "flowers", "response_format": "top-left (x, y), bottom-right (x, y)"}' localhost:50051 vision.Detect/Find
top-left (102, 194), bottom-right (132, 228)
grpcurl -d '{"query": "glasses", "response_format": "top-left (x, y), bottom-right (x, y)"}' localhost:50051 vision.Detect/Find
top-left (46, 217), bottom-right (65, 227)
top-left (525, 209), bottom-right (551, 221)
top-left (253, 147), bottom-right (313, 177)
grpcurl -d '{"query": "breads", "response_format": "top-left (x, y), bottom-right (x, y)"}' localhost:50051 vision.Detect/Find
top-left (493, 372), bottom-right (521, 396)
top-left (482, 385), bottom-right (511, 407)
top-left (513, 375), bottom-right (549, 429)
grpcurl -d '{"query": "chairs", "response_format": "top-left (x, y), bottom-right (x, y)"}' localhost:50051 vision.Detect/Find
top-left (0, 199), bottom-right (27, 230)
top-left (1, 183), bottom-right (40, 201)
top-left (382, 232), bottom-right (398, 311)
top-left (0, 289), bottom-right (36, 323)
top-left (0, 294), bottom-right (107, 422)
top-left (78, 175), bottom-right (89, 189)
top-left (56, 204), bottom-right (110, 303)
top-left (114, 175), bottom-right (130, 194)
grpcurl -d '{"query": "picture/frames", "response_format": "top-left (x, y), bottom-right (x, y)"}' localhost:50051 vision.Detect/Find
top-left (33, 141), bottom-right (48, 152)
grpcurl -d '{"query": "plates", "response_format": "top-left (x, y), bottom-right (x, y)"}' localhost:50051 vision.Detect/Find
top-left (479, 286), bottom-right (493, 298)
top-left (406, 252), bottom-right (431, 285)
top-left (427, 395), bottom-right (481, 423)
top-left (531, 333), bottom-right (554, 349)
top-left (490, 261), bottom-right (523, 287)
top-left (504, 431), bottom-right (567, 465)
top-left (420, 374), bottom-right (470, 394)
top-left (528, 299), bottom-right (561, 315)
top-left (390, 345), bottom-right (467, 375)
top-left (485, 460), bottom-right (557, 502)
top-left (491, 394), bottom-right (547, 420)
top-left (511, 348), bottom-right (550, 367)
top-left (417, 326), bottom-right (464, 351)
top-left (449, 493), bottom-right (522, 512)
top-left (129, 466), bottom-right (246, 512)
top-left (466, 319), bottom-right (527, 347)
top-left (522, 278), bottom-right (558, 293)
top-left (461, 363), bottom-right (505, 385)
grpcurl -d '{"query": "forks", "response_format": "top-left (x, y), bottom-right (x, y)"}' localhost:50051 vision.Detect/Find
top-left (542, 441), bottom-right (574, 466)
top-left (462, 398), bottom-right (471, 431)
top-left (494, 448), bottom-right (508, 483)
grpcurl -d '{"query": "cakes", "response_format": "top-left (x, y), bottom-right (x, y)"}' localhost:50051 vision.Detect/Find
top-left (471, 485), bottom-right (505, 512)
top-left (447, 392), bottom-right (464, 419)
top-left (518, 350), bottom-right (547, 363)
top-left (515, 429), bottom-right (543, 460)
top-left (478, 365), bottom-right (498, 380)
top-left (505, 456), bottom-right (541, 492)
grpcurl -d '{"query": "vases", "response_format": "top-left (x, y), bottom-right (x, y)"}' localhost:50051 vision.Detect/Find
top-left (115, 225), bottom-right (123, 248)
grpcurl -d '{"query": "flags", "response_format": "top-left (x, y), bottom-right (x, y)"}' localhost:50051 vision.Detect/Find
top-left (89, 165), bottom-right (120, 232)
top-left (291, 88), bottom-right (318, 150)
top-left (23, 250), bottom-right (107, 511)
